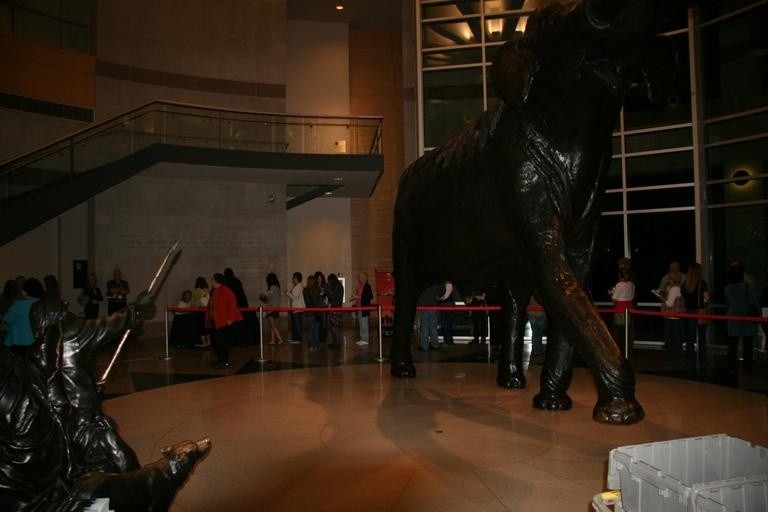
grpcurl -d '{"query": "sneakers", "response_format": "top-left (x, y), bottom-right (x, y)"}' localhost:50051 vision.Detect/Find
top-left (359, 340), bottom-right (368, 345)
top-left (356, 339), bottom-right (362, 343)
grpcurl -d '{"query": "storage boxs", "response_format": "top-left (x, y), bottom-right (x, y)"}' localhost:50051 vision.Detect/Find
top-left (693, 478), bottom-right (767, 511)
top-left (592, 489), bottom-right (626, 512)
top-left (604, 433), bottom-right (768, 511)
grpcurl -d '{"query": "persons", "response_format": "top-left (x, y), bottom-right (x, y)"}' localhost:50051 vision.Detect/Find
top-left (106, 269), bottom-right (130, 316)
top-left (437, 274), bottom-right (456, 347)
top-left (28, 294), bottom-right (151, 473)
top-left (82, 273), bottom-right (103, 318)
top-left (608, 255), bottom-right (711, 359)
top-left (418, 288), bottom-right (439, 351)
top-left (526, 296), bottom-right (547, 356)
top-left (0, 275), bottom-right (62, 359)
top-left (465, 291), bottom-right (489, 347)
top-left (169, 266), bottom-right (374, 368)
top-left (724, 261), bottom-right (760, 371)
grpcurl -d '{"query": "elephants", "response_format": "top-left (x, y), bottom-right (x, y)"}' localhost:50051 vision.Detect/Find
top-left (392, 0), bottom-right (677, 426)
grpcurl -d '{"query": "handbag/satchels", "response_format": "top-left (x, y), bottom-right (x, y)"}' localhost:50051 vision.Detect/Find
top-left (258, 293), bottom-right (269, 304)
